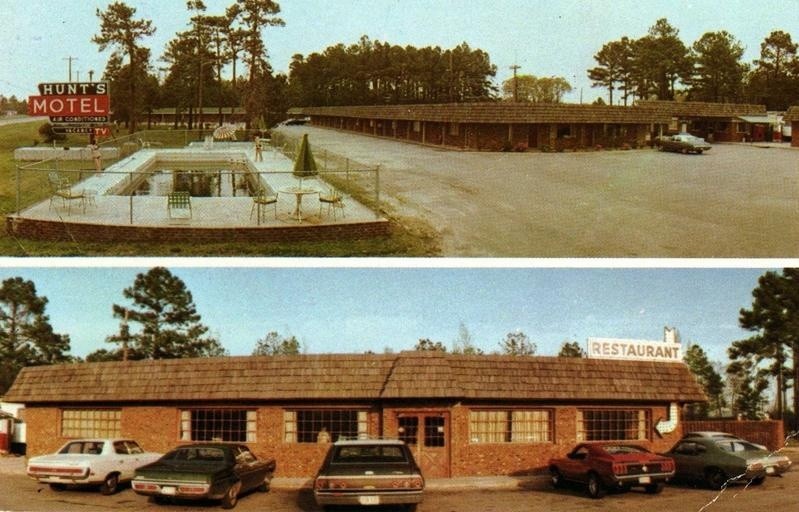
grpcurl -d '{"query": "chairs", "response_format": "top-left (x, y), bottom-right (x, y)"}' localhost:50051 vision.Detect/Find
top-left (78, 189), bottom-right (97, 208)
top-left (47, 172), bottom-right (68, 212)
top-left (319, 188), bottom-right (346, 221)
top-left (140, 138), bottom-right (150, 150)
top-left (189, 136), bottom-right (211, 151)
top-left (89, 448), bottom-right (101, 454)
top-left (249, 187), bottom-right (277, 224)
top-left (167, 191), bottom-right (193, 226)
top-left (58, 175), bottom-right (86, 216)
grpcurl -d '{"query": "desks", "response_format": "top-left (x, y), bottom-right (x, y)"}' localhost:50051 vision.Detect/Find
top-left (259, 139), bottom-right (272, 151)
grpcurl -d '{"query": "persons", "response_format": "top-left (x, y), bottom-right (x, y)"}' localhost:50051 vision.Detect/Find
top-left (87, 130), bottom-right (102, 178)
top-left (318, 426), bottom-right (332, 443)
top-left (253, 133), bottom-right (263, 162)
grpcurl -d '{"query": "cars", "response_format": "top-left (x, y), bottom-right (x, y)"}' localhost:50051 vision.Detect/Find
top-left (26, 437), bottom-right (168, 494)
top-left (661, 436), bottom-right (793, 488)
top-left (282, 118), bottom-right (307, 126)
top-left (666, 430), bottom-right (767, 452)
top-left (547, 441), bottom-right (676, 498)
top-left (129, 441), bottom-right (277, 509)
top-left (653, 133), bottom-right (711, 155)
top-left (310, 439), bottom-right (426, 510)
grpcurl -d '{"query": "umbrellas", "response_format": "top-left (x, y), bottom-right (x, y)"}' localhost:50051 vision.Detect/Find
top-left (213, 125), bottom-right (237, 149)
